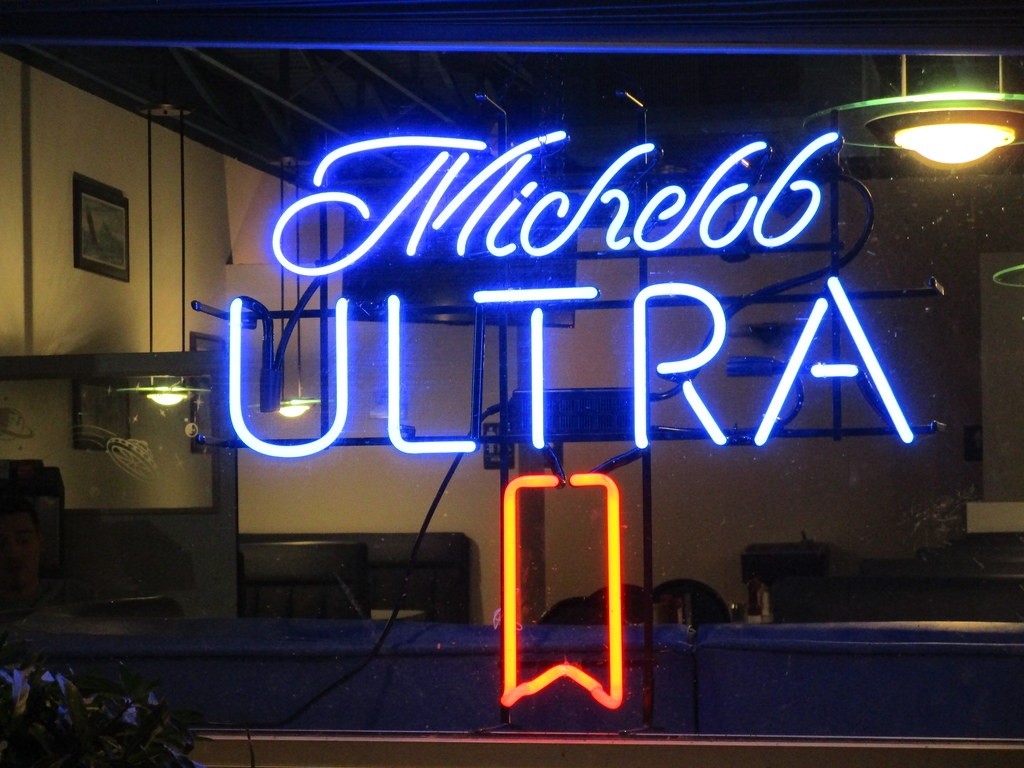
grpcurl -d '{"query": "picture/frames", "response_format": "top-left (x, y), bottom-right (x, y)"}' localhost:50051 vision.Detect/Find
top-left (72, 377), bottom-right (131, 452)
top-left (73, 177), bottom-right (130, 283)
top-left (189, 331), bottom-right (226, 391)
top-left (192, 394), bottom-right (213, 455)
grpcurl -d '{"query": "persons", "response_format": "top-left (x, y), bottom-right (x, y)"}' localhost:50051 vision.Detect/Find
top-left (0, 497), bottom-right (77, 620)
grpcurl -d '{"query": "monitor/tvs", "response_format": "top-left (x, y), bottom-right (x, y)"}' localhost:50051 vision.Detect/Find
top-left (238, 541), bottom-right (371, 621)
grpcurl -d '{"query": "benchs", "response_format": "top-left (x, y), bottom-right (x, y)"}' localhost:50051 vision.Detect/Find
top-left (247, 541), bottom-right (369, 620)
top-left (237, 534), bottom-right (484, 626)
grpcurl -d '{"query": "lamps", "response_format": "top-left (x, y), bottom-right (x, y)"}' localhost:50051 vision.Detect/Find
top-left (615, 88), bottom-right (645, 112)
top-left (473, 89), bottom-right (507, 121)
top-left (803, 54), bottom-right (1024, 166)
top-left (249, 153), bottom-right (334, 421)
top-left (117, 102), bottom-right (212, 408)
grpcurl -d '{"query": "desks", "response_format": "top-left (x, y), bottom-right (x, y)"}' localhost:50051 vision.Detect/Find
top-left (372, 609), bottom-right (425, 624)
